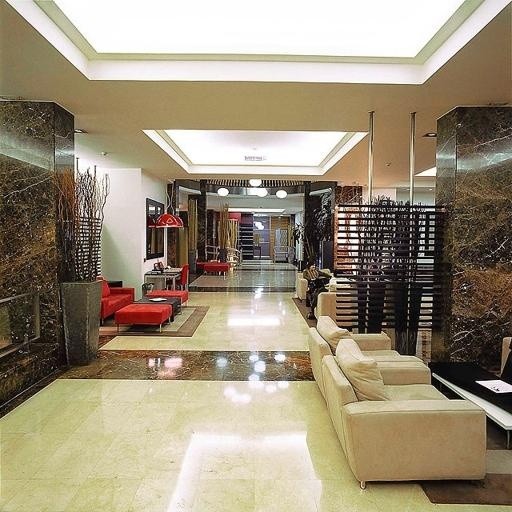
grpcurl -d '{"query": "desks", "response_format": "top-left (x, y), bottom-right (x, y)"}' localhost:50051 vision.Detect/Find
top-left (427, 360), bottom-right (512, 450)
top-left (253, 245), bottom-right (263, 259)
top-left (144, 267), bottom-right (189, 292)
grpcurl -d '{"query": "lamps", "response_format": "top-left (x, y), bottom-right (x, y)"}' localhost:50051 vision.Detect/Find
top-left (150, 177), bottom-right (185, 231)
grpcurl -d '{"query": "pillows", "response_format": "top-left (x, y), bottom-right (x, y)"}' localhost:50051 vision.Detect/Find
top-left (315, 315), bottom-right (352, 356)
top-left (334, 337), bottom-right (391, 401)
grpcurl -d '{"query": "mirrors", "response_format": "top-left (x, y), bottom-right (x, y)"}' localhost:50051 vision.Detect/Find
top-left (144, 199), bottom-right (167, 261)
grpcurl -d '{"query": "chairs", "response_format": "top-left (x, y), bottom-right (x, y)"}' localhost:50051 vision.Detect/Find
top-left (224, 248), bottom-right (240, 268)
top-left (166, 264), bottom-right (189, 291)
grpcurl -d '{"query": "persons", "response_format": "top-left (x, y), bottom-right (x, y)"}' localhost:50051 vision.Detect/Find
top-left (303, 260), bottom-right (332, 320)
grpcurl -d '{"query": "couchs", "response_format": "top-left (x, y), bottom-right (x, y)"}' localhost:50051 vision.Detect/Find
top-left (133, 296), bottom-right (183, 324)
top-left (145, 290), bottom-right (189, 315)
top-left (95, 276), bottom-right (136, 326)
top-left (317, 354), bottom-right (488, 489)
top-left (296, 268), bottom-right (387, 332)
top-left (304, 325), bottom-right (424, 407)
top-left (114, 304), bottom-right (173, 335)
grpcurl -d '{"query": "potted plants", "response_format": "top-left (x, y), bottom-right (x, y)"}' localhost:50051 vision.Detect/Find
top-left (47, 156), bottom-right (110, 366)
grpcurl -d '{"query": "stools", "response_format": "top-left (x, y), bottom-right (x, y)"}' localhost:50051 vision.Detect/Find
top-left (194, 259), bottom-right (231, 281)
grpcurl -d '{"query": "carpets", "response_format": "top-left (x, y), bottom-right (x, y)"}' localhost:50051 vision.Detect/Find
top-left (292, 297), bottom-right (318, 328)
top-left (417, 416), bottom-right (511, 506)
top-left (98, 305), bottom-right (210, 338)
